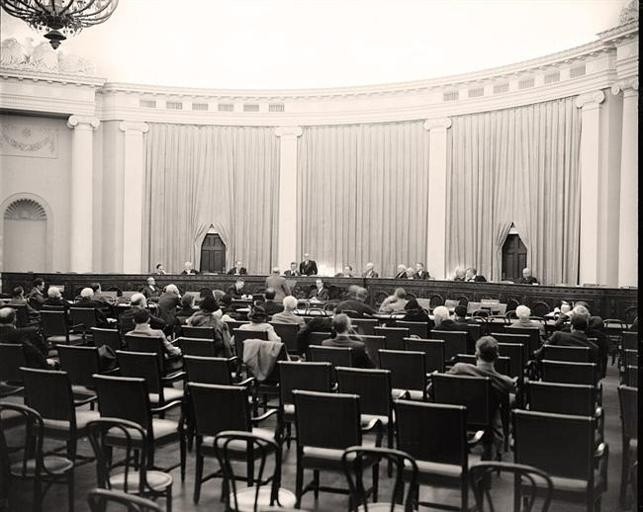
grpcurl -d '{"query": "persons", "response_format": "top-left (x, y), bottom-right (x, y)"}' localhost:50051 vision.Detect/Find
top-left (452, 266), bottom-right (466, 281)
top-left (230, 306), bottom-right (281, 345)
top-left (334, 265), bottom-right (353, 276)
top-left (125, 308), bottom-right (181, 358)
top-left (270, 295), bottom-right (305, 330)
top-left (255, 287), bottom-right (284, 314)
top-left (120, 294), bottom-right (164, 330)
top-left (379, 288), bottom-right (421, 315)
top-left (451, 305), bottom-right (472, 322)
top-left (175, 292), bottom-right (198, 322)
top-left (158, 283), bottom-right (181, 340)
top-left (333, 287), bottom-right (376, 319)
top-left (180, 261), bottom-right (198, 276)
top-left (360, 262), bottom-right (377, 277)
top-left (530, 313), bottom-right (600, 362)
top-left (309, 278), bottom-right (330, 300)
top-left (299, 254), bottom-right (317, 275)
top-left (10, 286), bottom-right (40, 323)
top-left (46, 283), bottom-right (68, 306)
top-left (433, 305), bottom-right (476, 357)
top-left (224, 277), bottom-right (252, 305)
top-left (187, 295), bottom-right (236, 367)
top-left (446, 336), bottom-right (518, 410)
top-left (411, 263), bottom-right (429, 279)
top-left (509, 305), bottom-right (545, 341)
top-left (394, 264), bottom-right (409, 278)
top-left (552, 305), bottom-right (604, 334)
top-left (321, 313), bottom-right (376, 369)
top-left (0, 308), bottom-right (58, 371)
top-left (226, 261), bottom-right (246, 276)
top-left (72, 287), bottom-right (111, 326)
top-left (142, 276), bottom-right (161, 304)
top-left (154, 263), bottom-right (166, 275)
top-left (265, 267), bottom-right (291, 300)
top-left (91, 282), bottom-right (117, 306)
top-left (29, 279), bottom-right (47, 312)
top-left (518, 267), bottom-right (537, 285)
top-left (464, 268), bottom-right (485, 282)
top-left (286, 262), bottom-right (298, 274)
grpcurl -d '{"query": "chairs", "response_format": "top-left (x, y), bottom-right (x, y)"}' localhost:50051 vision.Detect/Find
top-left (38, 309), bottom-right (91, 345)
top-left (512, 408), bottom-right (608, 510)
top-left (504, 324), bottom-right (558, 359)
top-left (86, 420), bottom-right (173, 512)
top-left (180, 326), bottom-right (229, 356)
top-left (0, 344), bottom-right (45, 398)
top-left (495, 343), bottom-right (528, 409)
top-left (378, 349), bottom-right (429, 401)
top-left (392, 397), bottom-right (486, 512)
top-left (467, 463), bottom-right (555, 512)
top-left (90, 373), bottom-right (187, 483)
top-left (456, 354), bottom-right (510, 377)
top-left (620, 365), bottom-right (640, 386)
top-left (307, 331), bottom-right (334, 347)
top-left (305, 346), bottom-right (354, 370)
top-left (331, 446), bottom-right (420, 512)
top-left (428, 373), bottom-right (504, 481)
top-left (56, 343), bottom-right (117, 409)
top-left (114, 349), bottom-right (184, 420)
top-left (430, 329), bottom-right (474, 361)
top-left (622, 346), bottom-right (638, 365)
top-left (268, 321), bottom-right (300, 362)
top-left (395, 319), bottom-right (428, 337)
top-left (334, 365), bottom-right (411, 477)
top-left (18, 365), bottom-right (111, 479)
top-left (292, 387), bottom-right (380, 512)
top-left (232, 327), bottom-right (270, 358)
top-left (374, 326), bottom-right (410, 350)
top-left (617, 384), bottom-right (641, 512)
top-left (529, 358), bottom-right (602, 410)
top-left (213, 430), bottom-right (298, 511)
top-left (181, 354), bottom-right (260, 453)
top-left (527, 381), bottom-right (606, 450)
top-left (352, 318), bottom-right (379, 336)
top-left (175, 337), bottom-right (218, 369)
top-left (490, 331), bottom-right (537, 381)
top-left (0, 402), bottom-right (77, 512)
top-left (350, 334), bottom-right (387, 369)
top-left (70, 306), bottom-right (99, 343)
top-left (402, 337), bottom-right (456, 373)
top-left (89, 326), bottom-right (124, 348)
top-left (603, 318), bottom-right (622, 345)
top-left (123, 334), bottom-right (185, 387)
top-left (277, 360), bottom-right (334, 449)
top-left (543, 345), bottom-right (591, 364)
top-left (622, 332), bottom-right (639, 346)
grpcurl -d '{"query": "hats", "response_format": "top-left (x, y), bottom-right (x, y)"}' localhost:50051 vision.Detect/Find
top-left (248, 306), bottom-right (268, 322)
top-left (47, 286), bottom-right (62, 298)
top-left (200, 296), bottom-right (220, 312)
top-left (405, 299), bottom-right (423, 312)
top-left (81, 287), bottom-right (95, 298)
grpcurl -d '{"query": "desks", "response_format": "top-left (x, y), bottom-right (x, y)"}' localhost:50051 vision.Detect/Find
top-left (0, 274), bottom-right (640, 321)
